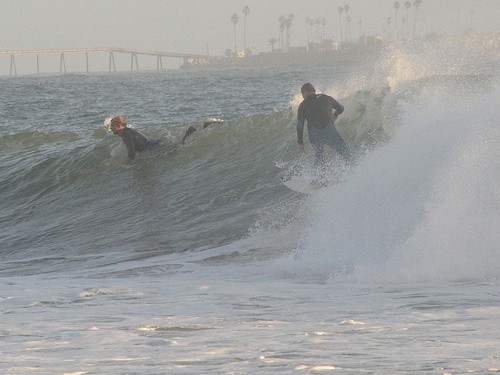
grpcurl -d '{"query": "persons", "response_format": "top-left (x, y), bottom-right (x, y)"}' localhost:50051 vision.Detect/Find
top-left (292, 82), bottom-right (357, 190)
top-left (110, 115), bottom-right (198, 165)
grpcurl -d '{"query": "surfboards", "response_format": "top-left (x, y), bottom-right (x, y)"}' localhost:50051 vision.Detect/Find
top-left (280, 175), bottom-right (327, 194)
